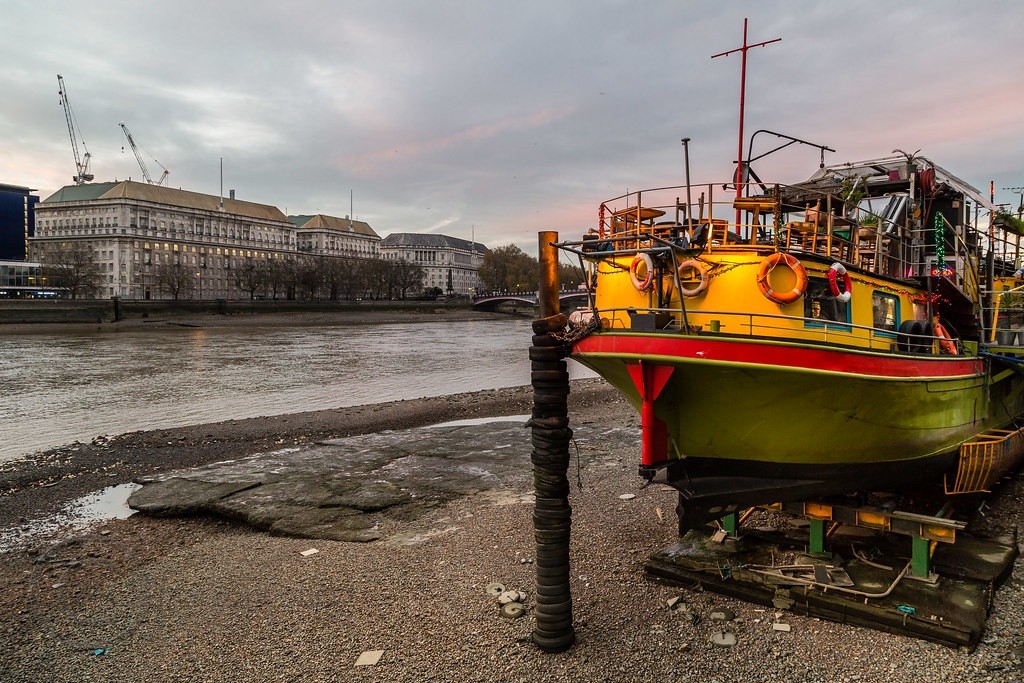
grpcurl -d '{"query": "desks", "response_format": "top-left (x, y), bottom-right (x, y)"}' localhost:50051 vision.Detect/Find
top-left (732, 196), bottom-right (775, 244)
top-left (611, 207), bottom-right (667, 250)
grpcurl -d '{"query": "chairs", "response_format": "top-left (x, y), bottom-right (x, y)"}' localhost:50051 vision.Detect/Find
top-left (651, 192), bottom-right (891, 275)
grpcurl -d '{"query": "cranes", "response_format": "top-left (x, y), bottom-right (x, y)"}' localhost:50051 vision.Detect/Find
top-left (55, 73), bottom-right (96, 186)
top-left (119, 120), bottom-right (171, 186)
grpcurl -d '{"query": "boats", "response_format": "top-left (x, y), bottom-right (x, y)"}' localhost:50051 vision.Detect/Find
top-left (548, 14), bottom-right (1024, 518)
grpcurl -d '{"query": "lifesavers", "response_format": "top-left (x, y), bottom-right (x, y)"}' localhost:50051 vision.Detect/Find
top-left (673, 260), bottom-right (707, 296)
top-left (933, 322), bottom-right (957, 356)
top-left (756, 253), bottom-right (807, 305)
top-left (630, 252), bottom-right (654, 289)
top-left (829, 262), bottom-right (852, 303)
top-left (897, 319), bottom-right (933, 354)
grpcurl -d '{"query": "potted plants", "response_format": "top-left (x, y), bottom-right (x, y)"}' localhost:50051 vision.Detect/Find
top-left (996, 293), bottom-right (1024, 346)
top-left (891, 149), bottom-right (936, 180)
